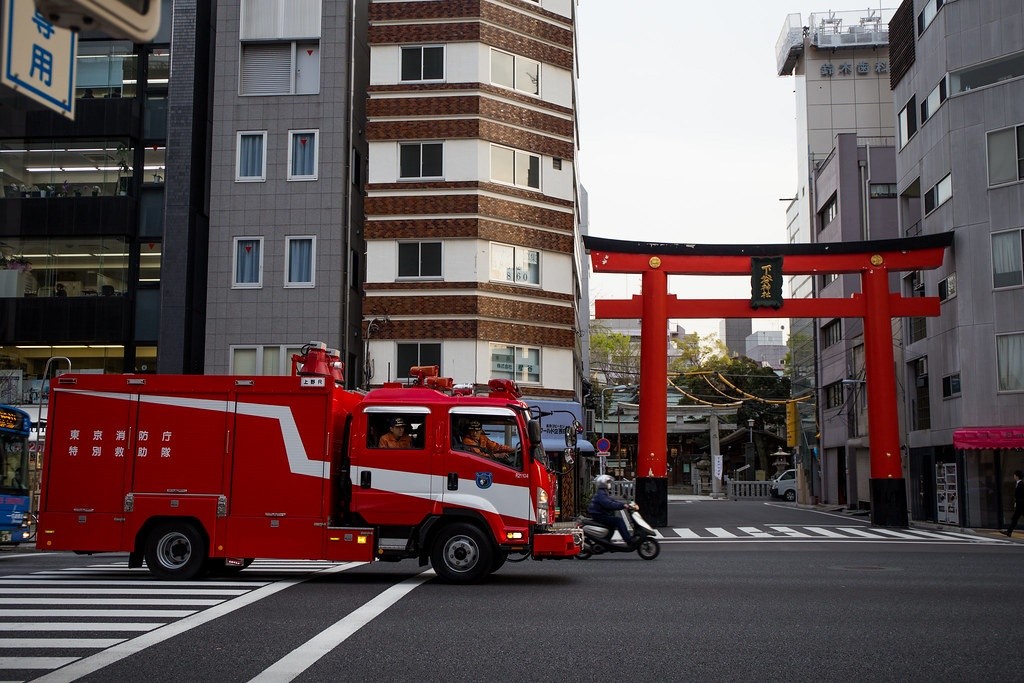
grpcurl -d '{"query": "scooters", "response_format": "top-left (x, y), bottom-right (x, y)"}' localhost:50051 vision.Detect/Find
top-left (567, 493), bottom-right (661, 560)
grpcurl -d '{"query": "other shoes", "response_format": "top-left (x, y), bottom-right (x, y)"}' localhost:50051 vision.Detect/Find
top-left (626, 536), bottom-right (639, 545)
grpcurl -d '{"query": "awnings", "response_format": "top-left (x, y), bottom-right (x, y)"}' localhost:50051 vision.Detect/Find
top-left (952, 426), bottom-right (1024, 450)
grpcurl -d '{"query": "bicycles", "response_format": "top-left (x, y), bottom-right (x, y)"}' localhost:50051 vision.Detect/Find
top-left (29, 514), bottom-right (39, 540)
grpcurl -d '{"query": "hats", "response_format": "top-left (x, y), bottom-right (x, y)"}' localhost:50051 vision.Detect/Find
top-left (467, 421), bottom-right (482, 430)
top-left (389, 417), bottom-right (407, 427)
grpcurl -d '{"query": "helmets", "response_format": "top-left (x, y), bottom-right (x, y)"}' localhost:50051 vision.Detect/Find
top-left (596, 474), bottom-right (615, 489)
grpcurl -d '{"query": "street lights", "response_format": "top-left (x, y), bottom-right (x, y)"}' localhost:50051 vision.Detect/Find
top-left (747, 417), bottom-right (755, 443)
top-left (601, 385), bottom-right (628, 474)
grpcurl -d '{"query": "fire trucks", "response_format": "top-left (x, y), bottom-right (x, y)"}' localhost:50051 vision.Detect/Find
top-left (35, 338), bottom-right (587, 582)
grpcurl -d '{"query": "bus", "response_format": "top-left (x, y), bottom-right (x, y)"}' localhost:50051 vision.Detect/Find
top-left (0, 404), bottom-right (37, 548)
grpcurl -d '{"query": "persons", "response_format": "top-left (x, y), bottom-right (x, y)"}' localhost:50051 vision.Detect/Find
top-left (378, 417), bottom-right (418, 449)
top-left (586, 475), bottom-right (640, 546)
top-left (998, 469), bottom-right (1024, 537)
top-left (462, 421), bottom-right (516, 459)
top-left (55, 283), bottom-right (67, 296)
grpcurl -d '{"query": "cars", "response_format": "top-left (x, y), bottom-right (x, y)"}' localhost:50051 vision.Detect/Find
top-left (770, 468), bottom-right (822, 502)
